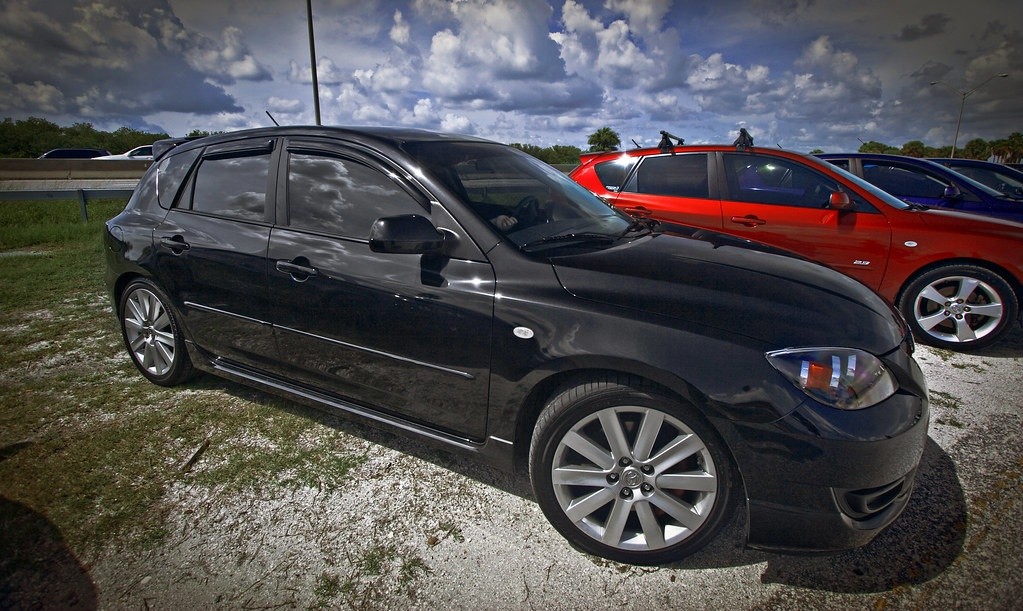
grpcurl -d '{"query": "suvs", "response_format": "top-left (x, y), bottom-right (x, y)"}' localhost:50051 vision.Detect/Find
top-left (566, 128), bottom-right (1023, 352)
top-left (105, 126), bottom-right (932, 566)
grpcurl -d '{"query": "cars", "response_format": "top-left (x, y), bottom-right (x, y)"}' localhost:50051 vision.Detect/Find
top-left (737, 152), bottom-right (1023, 225)
top-left (862, 157), bottom-right (1023, 200)
top-left (91, 144), bottom-right (155, 160)
top-left (33, 149), bottom-right (110, 159)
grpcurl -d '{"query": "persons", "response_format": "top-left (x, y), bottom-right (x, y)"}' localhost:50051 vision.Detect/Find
top-left (490, 215), bottom-right (518, 231)
